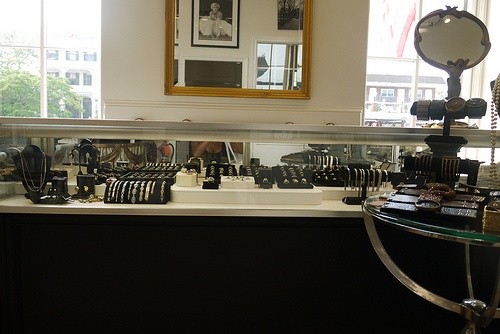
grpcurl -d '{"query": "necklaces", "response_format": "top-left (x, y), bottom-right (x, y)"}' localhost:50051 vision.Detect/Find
top-left (21, 151), bottom-right (44, 189)
top-left (486, 74), bottom-right (500, 191)
top-left (18, 150), bottom-right (47, 192)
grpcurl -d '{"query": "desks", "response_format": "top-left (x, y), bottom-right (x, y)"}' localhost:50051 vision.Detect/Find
top-left (361, 190), bottom-right (500, 334)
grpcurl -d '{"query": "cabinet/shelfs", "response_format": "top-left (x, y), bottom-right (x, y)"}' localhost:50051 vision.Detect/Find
top-left (0, 115), bottom-right (500, 334)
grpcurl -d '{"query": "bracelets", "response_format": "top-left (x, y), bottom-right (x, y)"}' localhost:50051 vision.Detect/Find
top-left (401, 155), bottom-right (461, 183)
top-left (306, 153), bottom-right (390, 195)
top-left (107, 161), bottom-right (184, 205)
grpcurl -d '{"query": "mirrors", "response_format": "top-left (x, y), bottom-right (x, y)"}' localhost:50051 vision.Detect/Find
top-left (410, 4), bottom-right (492, 74)
top-left (163, 0), bottom-right (312, 101)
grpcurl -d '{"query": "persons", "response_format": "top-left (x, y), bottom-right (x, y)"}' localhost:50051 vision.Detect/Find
top-left (199, 2), bottom-right (231, 39)
top-left (193, 141), bottom-right (234, 163)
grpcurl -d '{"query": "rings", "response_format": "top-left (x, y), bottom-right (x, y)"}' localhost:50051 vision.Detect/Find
top-left (204, 161), bottom-right (306, 187)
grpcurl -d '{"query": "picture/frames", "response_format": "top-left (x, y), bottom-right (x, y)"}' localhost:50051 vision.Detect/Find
top-left (190, 0), bottom-right (241, 49)
